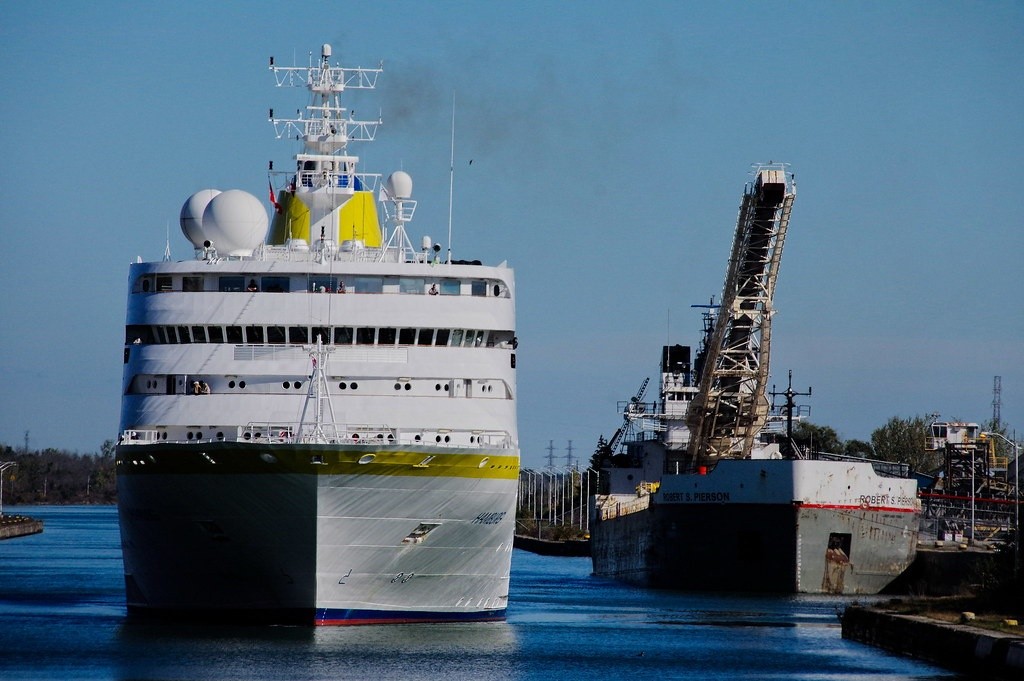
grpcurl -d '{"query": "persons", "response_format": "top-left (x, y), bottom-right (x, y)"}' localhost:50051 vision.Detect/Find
top-left (279, 431), bottom-right (286, 442)
top-left (428, 283), bottom-right (438, 295)
top-left (336, 281), bottom-right (346, 294)
top-left (354, 434), bottom-right (359, 444)
top-left (193, 381), bottom-right (210, 395)
top-left (133, 336), bottom-right (142, 343)
top-left (247, 279), bottom-right (258, 292)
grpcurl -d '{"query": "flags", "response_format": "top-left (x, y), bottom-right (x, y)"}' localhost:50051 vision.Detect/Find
top-left (269, 177), bottom-right (283, 215)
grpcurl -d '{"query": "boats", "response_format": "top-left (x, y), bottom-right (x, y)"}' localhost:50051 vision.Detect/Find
top-left (113, 44), bottom-right (522, 629)
top-left (588, 161), bottom-right (920, 601)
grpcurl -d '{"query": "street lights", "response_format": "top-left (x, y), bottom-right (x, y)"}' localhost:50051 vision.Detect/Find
top-left (525, 468), bottom-right (537, 520)
top-left (521, 468), bottom-right (531, 513)
top-left (533, 469), bottom-right (543, 520)
top-left (542, 467), bottom-right (557, 526)
top-left (563, 466), bottom-right (582, 530)
top-left (981, 430), bottom-right (1021, 533)
top-left (555, 464), bottom-right (573, 529)
top-left (568, 464), bottom-right (589, 532)
top-left (540, 469), bottom-right (551, 525)
top-left (548, 466), bottom-right (564, 526)
top-left (581, 464), bottom-right (599, 495)
